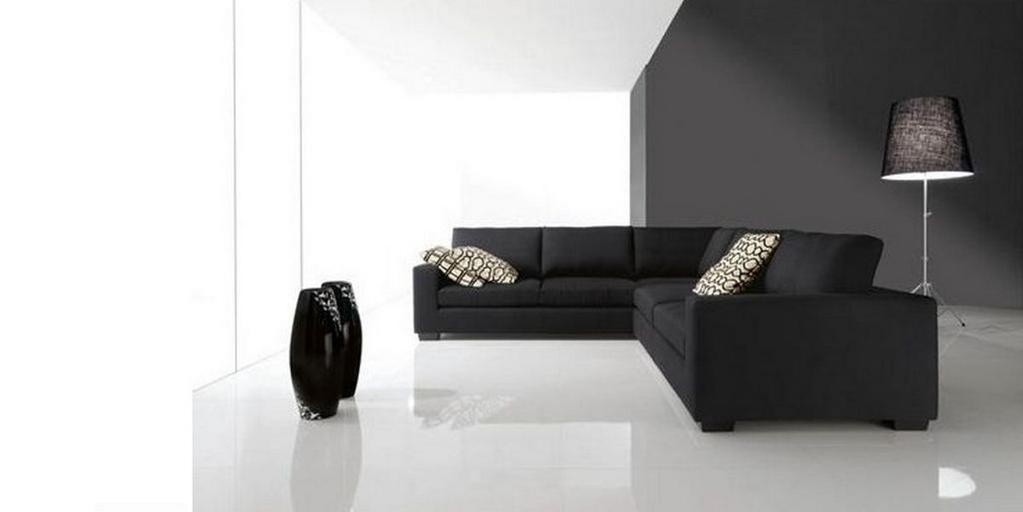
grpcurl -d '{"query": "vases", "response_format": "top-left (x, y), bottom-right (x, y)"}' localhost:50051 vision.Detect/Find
top-left (290, 289), bottom-right (343, 420)
top-left (321, 280), bottom-right (362, 398)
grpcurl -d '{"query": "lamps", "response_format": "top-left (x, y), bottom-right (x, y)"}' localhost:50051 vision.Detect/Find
top-left (879, 96), bottom-right (978, 331)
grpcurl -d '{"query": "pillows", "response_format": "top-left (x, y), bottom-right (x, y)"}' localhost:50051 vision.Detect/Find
top-left (692, 232), bottom-right (782, 297)
top-left (419, 245), bottom-right (485, 287)
top-left (448, 245), bottom-right (518, 284)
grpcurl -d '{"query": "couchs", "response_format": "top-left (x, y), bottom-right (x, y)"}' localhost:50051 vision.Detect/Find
top-left (413, 262), bottom-right (938, 431)
